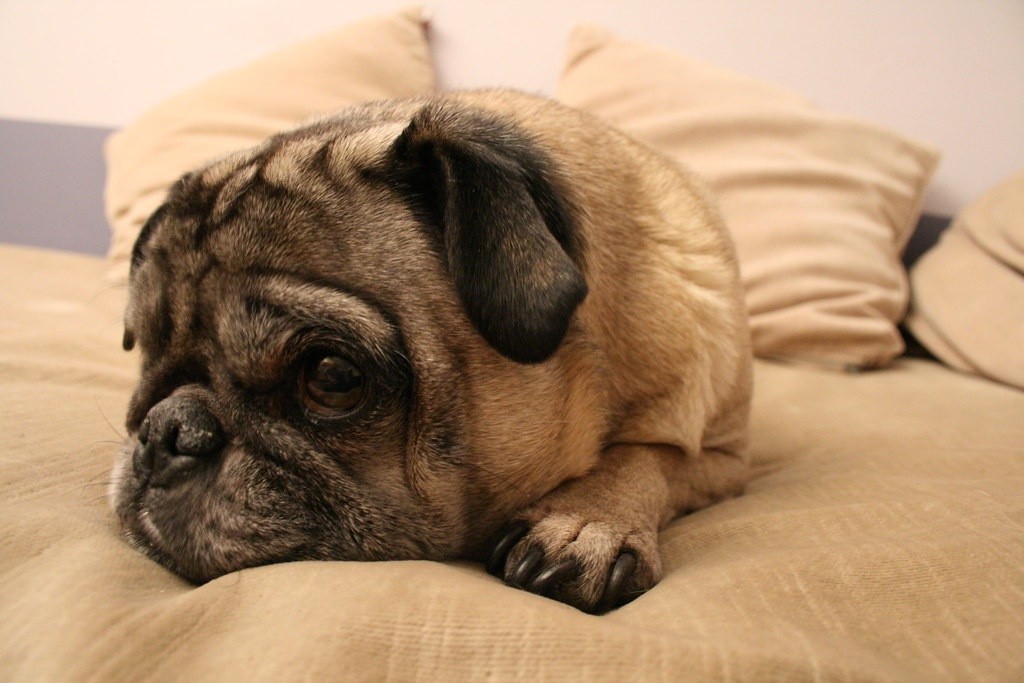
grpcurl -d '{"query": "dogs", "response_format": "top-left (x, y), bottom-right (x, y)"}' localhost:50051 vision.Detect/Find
top-left (102, 89), bottom-right (755, 618)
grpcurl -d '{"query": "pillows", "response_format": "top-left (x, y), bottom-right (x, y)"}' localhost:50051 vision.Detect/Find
top-left (904, 163), bottom-right (1024, 390)
top-left (102, 5), bottom-right (439, 289)
top-left (531, 23), bottom-right (939, 377)
